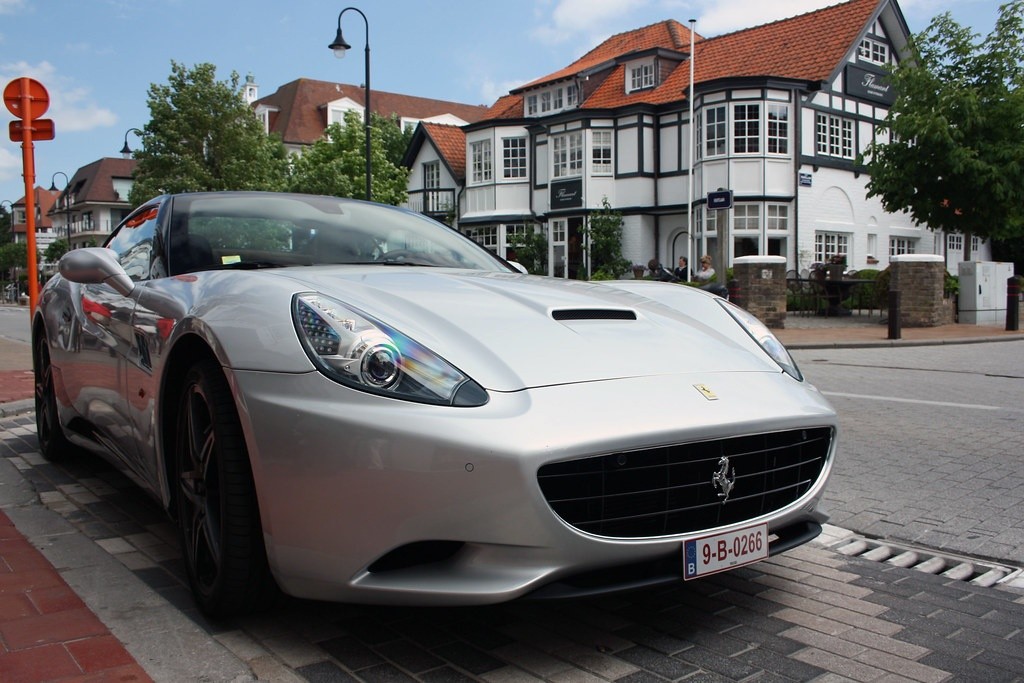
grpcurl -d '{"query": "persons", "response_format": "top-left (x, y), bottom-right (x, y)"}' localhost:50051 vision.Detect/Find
top-left (693, 256), bottom-right (715, 280)
top-left (72, 318), bottom-right (83, 354)
top-left (674, 257), bottom-right (694, 282)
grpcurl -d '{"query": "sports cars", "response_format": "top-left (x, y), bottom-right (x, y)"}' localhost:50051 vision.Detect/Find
top-left (30, 189), bottom-right (839, 631)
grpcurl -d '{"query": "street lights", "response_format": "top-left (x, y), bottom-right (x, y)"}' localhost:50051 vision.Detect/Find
top-left (327, 6), bottom-right (372, 202)
top-left (119, 128), bottom-right (146, 158)
top-left (48, 171), bottom-right (71, 251)
top-left (0, 200), bottom-right (16, 283)
